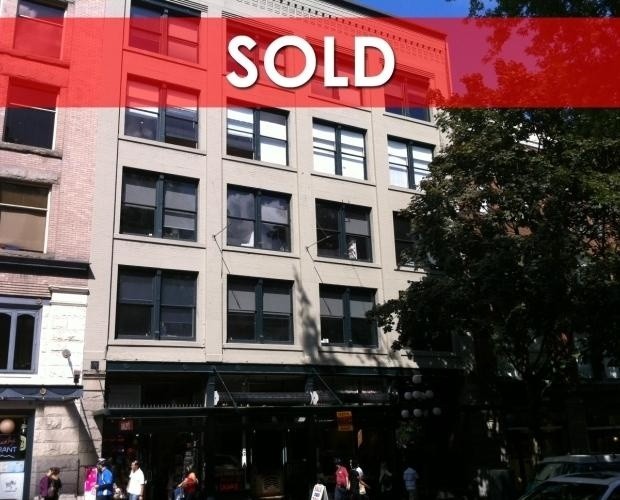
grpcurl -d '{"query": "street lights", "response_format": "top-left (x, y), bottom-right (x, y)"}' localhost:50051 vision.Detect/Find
top-left (401, 374), bottom-right (442, 422)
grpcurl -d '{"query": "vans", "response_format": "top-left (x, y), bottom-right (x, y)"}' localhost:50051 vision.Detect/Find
top-left (529, 452), bottom-right (620, 489)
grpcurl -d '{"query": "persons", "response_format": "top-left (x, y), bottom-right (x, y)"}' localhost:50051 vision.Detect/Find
top-left (39, 458), bottom-right (196, 500)
top-left (309, 457), bottom-right (489, 500)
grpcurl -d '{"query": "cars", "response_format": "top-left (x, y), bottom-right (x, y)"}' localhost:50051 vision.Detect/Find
top-left (521, 471), bottom-right (620, 500)
